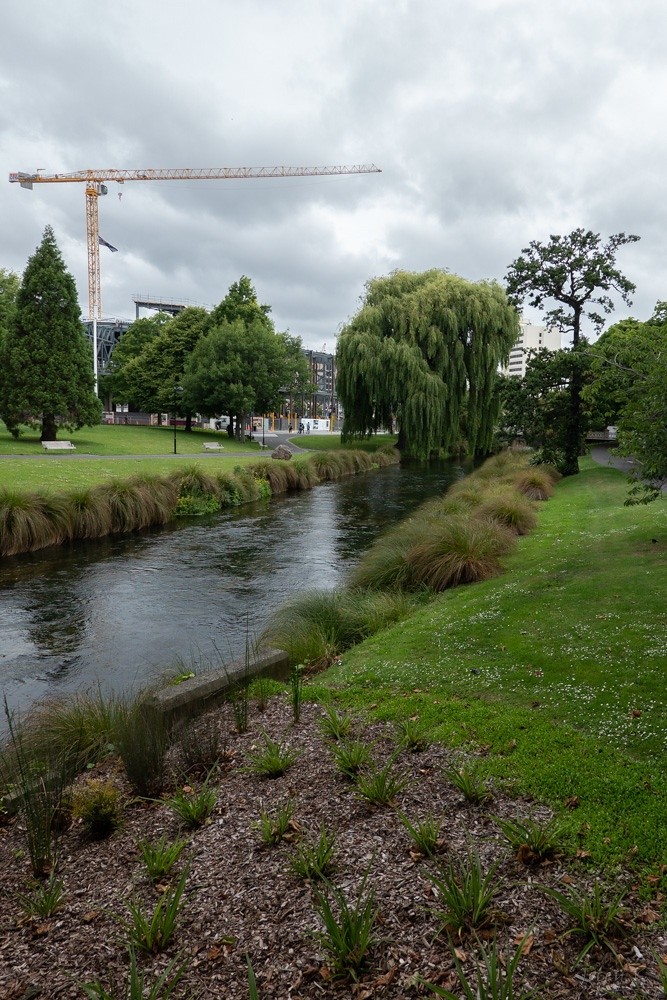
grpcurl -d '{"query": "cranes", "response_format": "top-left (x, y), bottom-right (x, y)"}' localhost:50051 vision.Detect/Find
top-left (8, 162), bottom-right (382, 321)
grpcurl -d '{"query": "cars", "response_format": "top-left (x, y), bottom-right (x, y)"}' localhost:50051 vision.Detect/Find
top-left (215, 416), bottom-right (241, 431)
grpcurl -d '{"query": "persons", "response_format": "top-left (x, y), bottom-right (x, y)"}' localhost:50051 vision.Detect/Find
top-left (297, 422), bottom-right (304, 434)
top-left (288, 422), bottom-right (293, 433)
top-left (325, 407), bottom-right (330, 419)
top-left (306, 422), bottom-right (310, 434)
